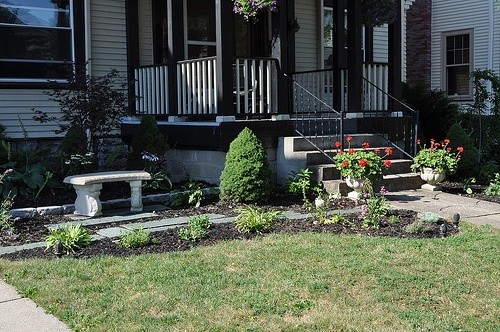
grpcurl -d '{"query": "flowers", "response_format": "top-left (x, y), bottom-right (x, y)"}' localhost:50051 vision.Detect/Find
top-left (409, 138), bottom-right (463, 174)
top-left (332, 136), bottom-right (394, 179)
top-left (231, 0), bottom-right (280, 24)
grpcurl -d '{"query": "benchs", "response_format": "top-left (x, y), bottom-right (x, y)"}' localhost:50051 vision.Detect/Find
top-left (62, 169), bottom-right (152, 218)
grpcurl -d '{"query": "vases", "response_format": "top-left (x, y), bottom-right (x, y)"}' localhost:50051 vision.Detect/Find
top-left (344, 177), bottom-right (369, 199)
top-left (419, 167), bottom-right (446, 191)
